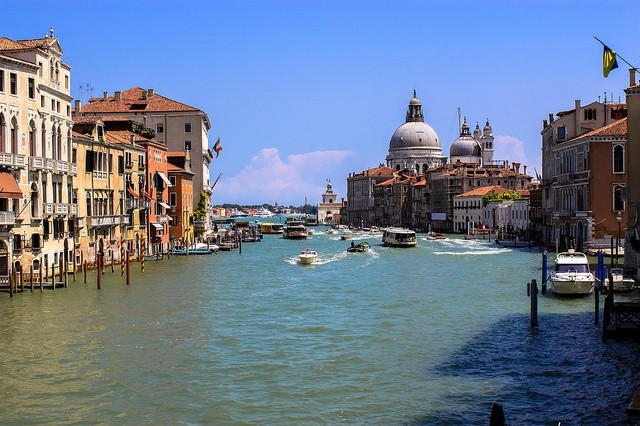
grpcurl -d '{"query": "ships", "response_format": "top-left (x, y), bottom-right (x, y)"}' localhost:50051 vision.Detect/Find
top-left (283, 215), bottom-right (309, 238)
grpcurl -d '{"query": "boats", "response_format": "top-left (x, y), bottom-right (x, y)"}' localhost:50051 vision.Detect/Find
top-left (324, 226), bottom-right (334, 234)
top-left (285, 216), bottom-right (318, 226)
top-left (166, 243), bottom-right (219, 254)
top-left (346, 240), bottom-right (371, 253)
top-left (499, 240), bottom-right (536, 248)
top-left (369, 226), bottom-right (380, 235)
top-left (308, 228), bottom-right (315, 235)
top-left (381, 225), bottom-right (393, 231)
top-left (298, 246), bottom-right (318, 264)
top-left (549, 248), bottom-right (593, 295)
top-left (426, 229), bottom-right (444, 239)
top-left (261, 222), bottom-right (283, 233)
top-left (382, 225), bottom-right (418, 248)
top-left (341, 227), bottom-right (352, 240)
top-left (595, 264), bottom-right (635, 291)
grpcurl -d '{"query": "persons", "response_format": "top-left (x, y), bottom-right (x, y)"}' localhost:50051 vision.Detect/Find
top-left (351, 240), bottom-right (354, 248)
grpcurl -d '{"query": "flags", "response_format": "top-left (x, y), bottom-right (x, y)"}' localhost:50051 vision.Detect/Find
top-left (213, 137), bottom-right (223, 159)
top-left (603, 45), bottom-right (618, 78)
